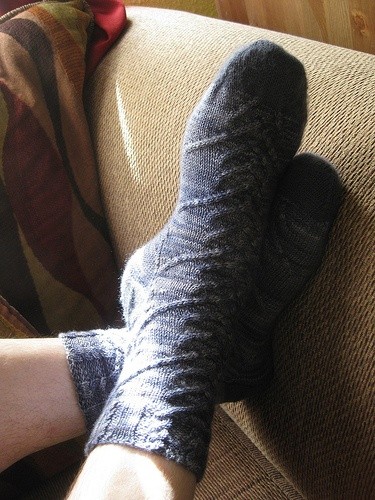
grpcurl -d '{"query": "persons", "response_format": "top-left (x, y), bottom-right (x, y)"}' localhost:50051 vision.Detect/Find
top-left (0, 39), bottom-right (347, 500)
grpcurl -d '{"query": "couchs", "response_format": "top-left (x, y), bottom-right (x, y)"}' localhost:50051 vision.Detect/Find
top-left (1, 1), bottom-right (374, 500)
